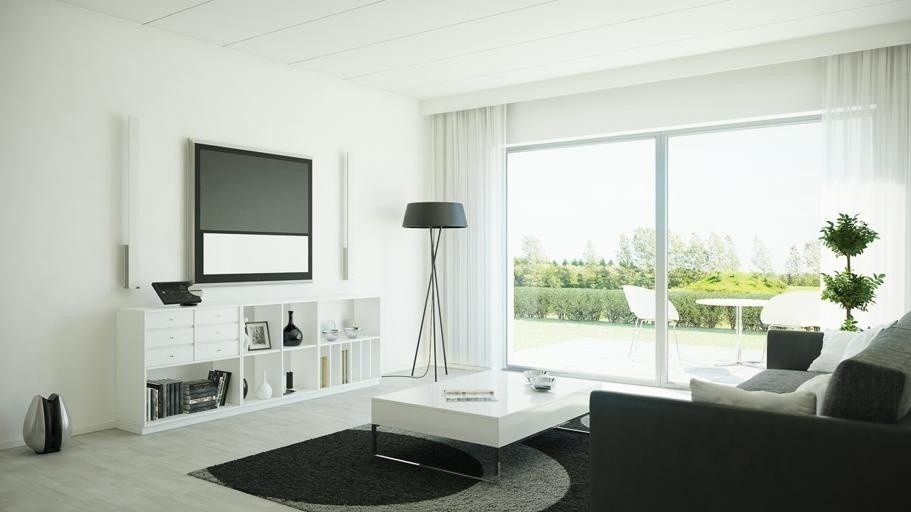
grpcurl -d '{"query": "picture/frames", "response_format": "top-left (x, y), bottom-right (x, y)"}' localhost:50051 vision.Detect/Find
top-left (243, 320), bottom-right (272, 351)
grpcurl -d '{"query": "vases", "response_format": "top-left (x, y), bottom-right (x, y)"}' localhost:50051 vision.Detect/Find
top-left (258, 370), bottom-right (273, 399)
top-left (283, 310), bottom-right (303, 346)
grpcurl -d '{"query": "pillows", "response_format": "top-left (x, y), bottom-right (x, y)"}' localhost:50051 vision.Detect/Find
top-left (691, 374), bottom-right (831, 414)
top-left (800, 319), bottom-right (899, 372)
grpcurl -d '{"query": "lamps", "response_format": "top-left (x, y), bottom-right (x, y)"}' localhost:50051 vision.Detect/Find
top-left (398, 199), bottom-right (469, 381)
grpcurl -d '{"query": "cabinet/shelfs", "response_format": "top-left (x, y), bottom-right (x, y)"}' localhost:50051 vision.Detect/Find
top-left (119, 293), bottom-right (382, 436)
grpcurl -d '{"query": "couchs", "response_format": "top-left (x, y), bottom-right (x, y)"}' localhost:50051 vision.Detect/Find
top-left (589, 310), bottom-right (911, 510)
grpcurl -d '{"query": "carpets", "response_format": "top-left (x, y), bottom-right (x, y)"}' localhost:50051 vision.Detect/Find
top-left (182, 412), bottom-right (589, 511)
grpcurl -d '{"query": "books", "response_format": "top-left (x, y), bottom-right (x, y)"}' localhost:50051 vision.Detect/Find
top-left (147, 370), bottom-right (232, 421)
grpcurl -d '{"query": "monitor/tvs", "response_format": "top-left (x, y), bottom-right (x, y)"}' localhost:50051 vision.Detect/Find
top-left (188, 137), bottom-right (316, 287)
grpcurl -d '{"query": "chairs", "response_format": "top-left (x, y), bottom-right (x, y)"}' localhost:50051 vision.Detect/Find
top-left (623, 283), bottom-right (682, 366)
top-left (759, 289), bottom-right (822, 331)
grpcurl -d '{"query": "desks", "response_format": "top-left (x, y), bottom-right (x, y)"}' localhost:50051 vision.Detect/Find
top-left (694, 294), bottom-right (765, 369)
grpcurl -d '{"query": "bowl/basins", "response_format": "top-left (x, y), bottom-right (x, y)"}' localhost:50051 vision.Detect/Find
top-left (343, 327), bottom-right (362, 338)
top-left (321, 328), bottom-right (342, 342)
